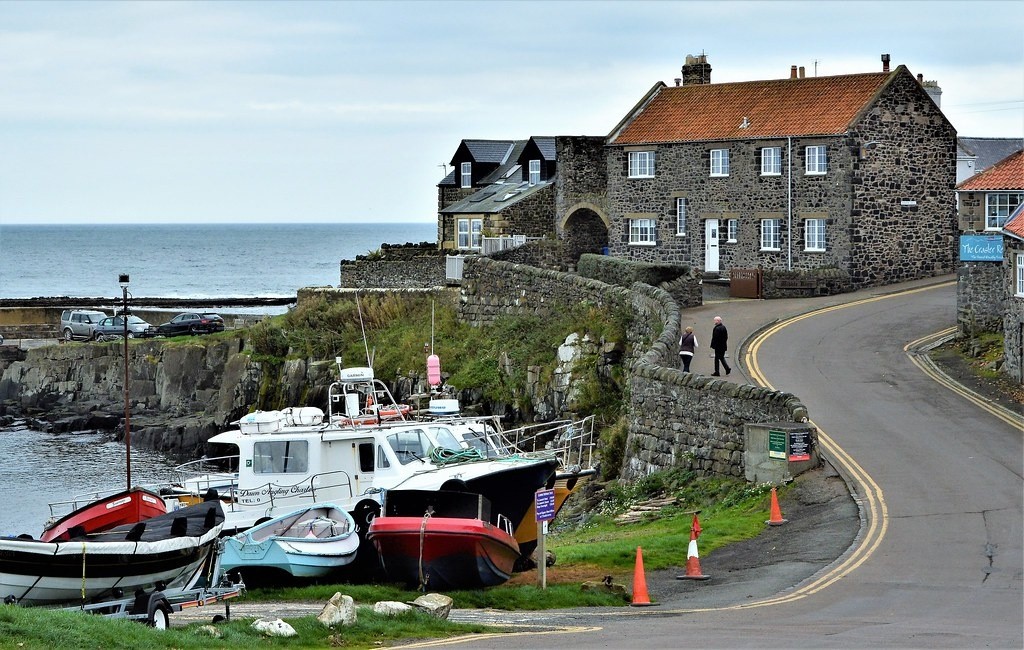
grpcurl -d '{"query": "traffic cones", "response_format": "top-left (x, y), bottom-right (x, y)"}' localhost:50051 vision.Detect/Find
top-left (631, 546), bottom-right (661, 606)
top-left (765, 487), bottom-right (788, 526)
top-left (676, 527), bottom-right (711, 580)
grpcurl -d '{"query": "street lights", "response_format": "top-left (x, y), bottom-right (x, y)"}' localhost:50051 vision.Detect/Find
top-left (118, 272), bottom-right (133, 491)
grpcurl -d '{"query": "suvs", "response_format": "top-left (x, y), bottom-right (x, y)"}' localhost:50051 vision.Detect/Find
top-left (157, 313), bottom-right (226, 336)
top-left (60, 309), bottom-right (112, 340)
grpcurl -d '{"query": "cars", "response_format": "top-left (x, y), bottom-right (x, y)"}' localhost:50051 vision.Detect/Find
top-left (92, 314), bottom-right (156, 340)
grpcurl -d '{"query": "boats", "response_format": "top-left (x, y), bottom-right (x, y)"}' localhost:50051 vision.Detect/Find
top-left (367, 489), bottom-right (522, 590)
top-left (172, 300), bottom-right (596, 573)
top-left (0, 488), bottom-right (229, 606)
top-left (157, 292), bottom-right (562, 538)
top-left (220, 502), bottom-right (360, 588)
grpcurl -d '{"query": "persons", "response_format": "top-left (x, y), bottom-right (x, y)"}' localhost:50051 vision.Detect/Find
top-left (678, 326), bottom-right (699, 372)
top-left (710, 316), bottom-right (731, 376)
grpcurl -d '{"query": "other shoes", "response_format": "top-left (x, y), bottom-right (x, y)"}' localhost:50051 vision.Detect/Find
top-left (711, 372), bottom-right (720, 376)
top-left (726, 368), bottom-right (731, 375)
